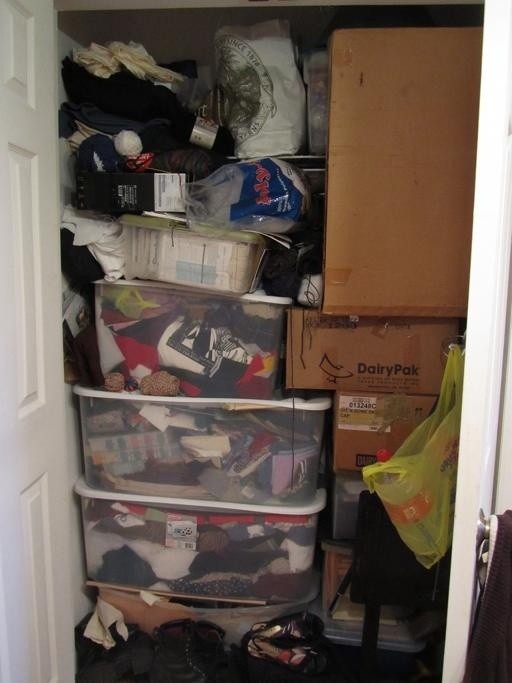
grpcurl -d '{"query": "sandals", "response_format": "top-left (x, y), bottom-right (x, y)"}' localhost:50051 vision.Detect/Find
top-left (241, 611), bottom-right (323, 650)
top-left (246, 638), bottom-right (326, 675)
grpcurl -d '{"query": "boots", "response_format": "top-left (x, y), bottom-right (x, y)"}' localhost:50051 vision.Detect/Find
top-left (193, 621), bottom-right (236, 678)
top-left (151, 618), bottom-right (233, 683)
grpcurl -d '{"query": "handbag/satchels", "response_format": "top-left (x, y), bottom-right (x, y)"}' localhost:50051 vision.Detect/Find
top-left (211, 18), bottom-right (308, 159)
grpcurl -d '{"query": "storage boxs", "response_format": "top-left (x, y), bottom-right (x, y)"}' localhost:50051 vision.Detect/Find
top-left (94, 589), bottom-right (319, 648)
top-left (304, 25), bottom-right (480, 319)
top-left (331, 390), bottom-right (439, 470)
top-left (92, 278), bottom-right (293, 401)
top-left (71, 380), bottom-right (331, 508)
top-left (72, 474), bottom-right (328, 603)
top-left (281, 306), bottom-right (464, 396)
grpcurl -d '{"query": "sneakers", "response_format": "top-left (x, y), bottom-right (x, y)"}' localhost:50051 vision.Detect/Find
top-left (75, 611), bottom-right (154, 683)
